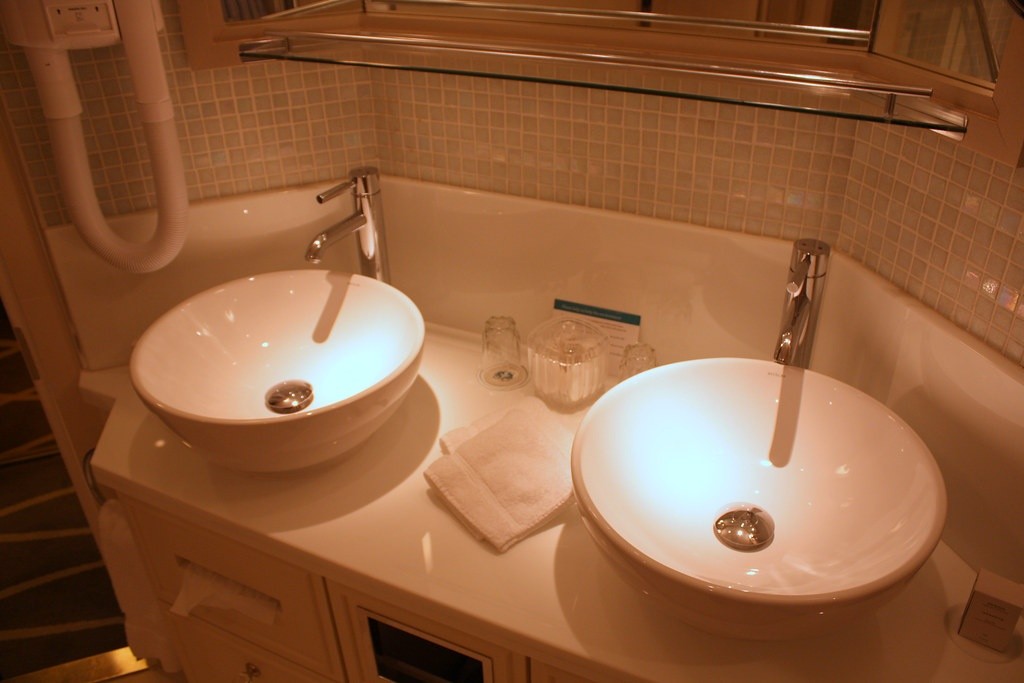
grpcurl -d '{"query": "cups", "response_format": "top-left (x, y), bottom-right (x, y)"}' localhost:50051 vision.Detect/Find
top-left (527, 316), bottom-right (609, 408)
top-left (616, 344), bottom-right (655, 382)
top-left (483, 316), bottom-right (520, 386)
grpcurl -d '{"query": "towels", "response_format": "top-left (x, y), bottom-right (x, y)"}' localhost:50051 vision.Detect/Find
top-left (423, 393), bottom-right (578, 546)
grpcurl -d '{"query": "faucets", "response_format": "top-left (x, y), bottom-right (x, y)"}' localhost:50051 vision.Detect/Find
top-left (774, 237), bottom-right (833, 367)
top-left (304, 165), bottom-right (391, 280)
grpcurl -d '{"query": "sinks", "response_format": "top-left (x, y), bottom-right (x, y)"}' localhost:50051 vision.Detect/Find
top-left (129, 267), bottom-right (428, 477)
top-left (572, 353), bottom-right (950, 601)
